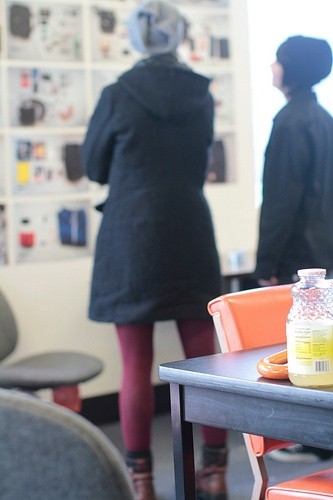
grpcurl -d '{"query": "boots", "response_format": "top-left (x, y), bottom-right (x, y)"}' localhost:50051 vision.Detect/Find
top-left (124, 449), bottom-right (156, 500)
top-left (195, 445), bottom-right (229, 500)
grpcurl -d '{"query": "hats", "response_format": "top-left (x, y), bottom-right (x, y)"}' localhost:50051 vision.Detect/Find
top-left (127, 1), bottom-right (186, 55)
top-left (277, 35), bottom-right (333, 88)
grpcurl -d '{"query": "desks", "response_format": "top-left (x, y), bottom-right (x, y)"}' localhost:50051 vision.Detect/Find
top-left (159, 341), bottom-right (333, 500)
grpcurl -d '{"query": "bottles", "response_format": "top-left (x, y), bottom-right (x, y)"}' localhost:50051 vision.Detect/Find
top-left (17, 218), bottom-right (36, 262)
top-left (285, 268), bottom-right (333, 386)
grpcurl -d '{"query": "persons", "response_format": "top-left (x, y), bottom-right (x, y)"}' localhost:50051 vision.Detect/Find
top-left (255, 35), bottom-right (333, 464)
top-left (81, 0), bottom-right (228, 500)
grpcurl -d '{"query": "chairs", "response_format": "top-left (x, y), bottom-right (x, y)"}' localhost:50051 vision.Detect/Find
top-left (0, 389), bottom-right (135, 500)
top-left (208, 280), bottom-right (333, 500)
top-left (0, 289), bottom-right (104, 396)
